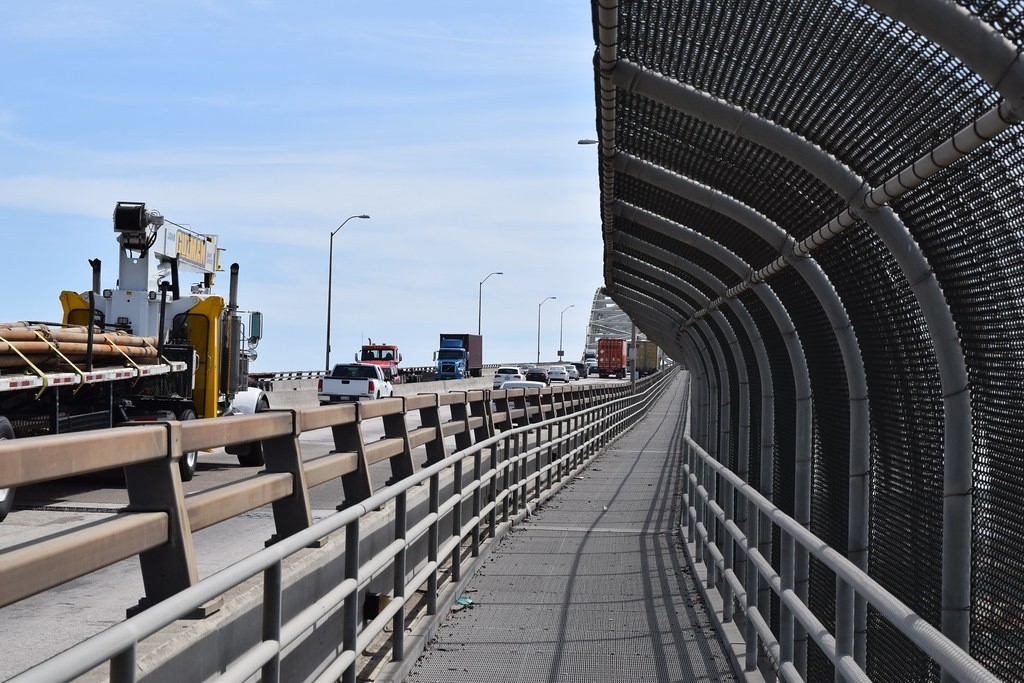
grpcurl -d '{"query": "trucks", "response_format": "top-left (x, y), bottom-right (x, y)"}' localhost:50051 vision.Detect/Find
top-left (597, 339), bottom-right (628, 379)
top-left (635, 340), bottom-right (662, 377)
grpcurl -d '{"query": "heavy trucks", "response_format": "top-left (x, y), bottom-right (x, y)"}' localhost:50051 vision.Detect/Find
top-left (355, 337), bottom-right (424, 384)
top-left (0, 200), bottom-right (271, 523)
top-left (433, 333), bottom-right (482, 381)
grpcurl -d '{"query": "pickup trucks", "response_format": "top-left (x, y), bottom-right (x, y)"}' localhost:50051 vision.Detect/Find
top-left (317, 362), bottom-right (394, 406)
top-left (585, 359), bottom-right (598, 371)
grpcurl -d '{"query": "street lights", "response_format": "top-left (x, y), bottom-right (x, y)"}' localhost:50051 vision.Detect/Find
top-left (325, 214), bottom-right (370, 376)
top-left (537, 297), bottom-right (557, 365)
top-left (479, 272), bottom-right (503, 337)
top-left (559, 305), bottom-right (574, 362)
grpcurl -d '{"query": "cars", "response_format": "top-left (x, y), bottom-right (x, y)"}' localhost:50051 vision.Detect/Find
top-left (565, 365), bottom-right (581, 381)
top-left (572, 362), bottom-right (588, 378)
top-left (491, 381), bottom-right (554, 429)
top-left (547, 366), bottom-right (570, 383)
top-left (523, 368), bottom-right (551, 386)
top-left (493, 367), bottom-right (527, 392)
